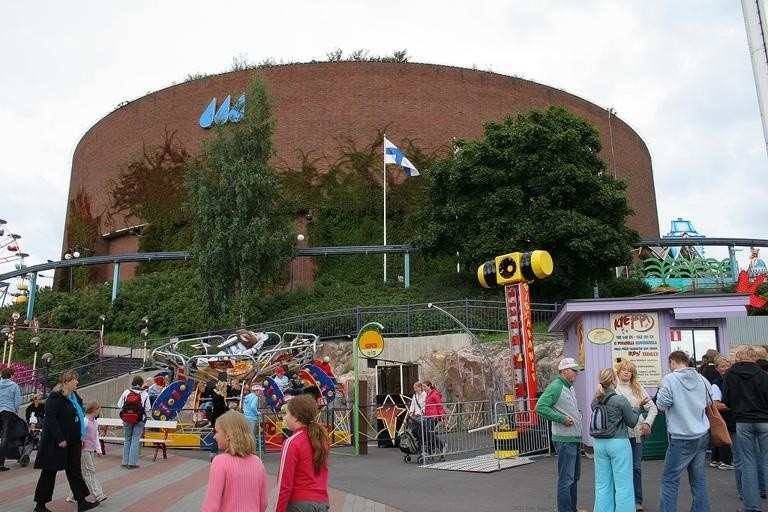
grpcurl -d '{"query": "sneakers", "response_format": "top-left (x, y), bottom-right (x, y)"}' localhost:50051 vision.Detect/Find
top-left (78, 501), bottom-right (99, 512)
top-left (636, 503), bottom-right (643, 510)
top-left (441, 443), bottom-right (448, 454)
top-left (710, 461), bottom-right (719, 467)
top-left (719, 461), bottom-right (734, 469)
top-left (95, 494), bottom-right (107, 501)
top-left (121, 464), bottom-right (128, 468)
top-left (33, 506), bottom-right (51, 512)
top-left (0, 466), bottom-right (9, 470)
top-left (128, 465), bottom-right (139, 468)
top-left (66, 497), bottom-right (77, 502)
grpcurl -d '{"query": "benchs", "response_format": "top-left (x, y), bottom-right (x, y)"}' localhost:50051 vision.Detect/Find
top-left (96, 416), bottom-right (178, 463)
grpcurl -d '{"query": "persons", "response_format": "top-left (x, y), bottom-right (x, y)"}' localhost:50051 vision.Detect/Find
top-left (407, 381), bottom-right (429, 444)
top-left (650, 345), bottom-right (767, 512)
top-left (1, 365), bottom-right (173, 512)
top-left (419, 381), bottom-right (448, 460)
top-left (611, 358), bottom-right (660, 511)
top-left (586, 367), bottom-right (653, 512)
top-left (534, 356), bottom-right (590, 512)
top-left (197, 367), bottom-right (332, 512)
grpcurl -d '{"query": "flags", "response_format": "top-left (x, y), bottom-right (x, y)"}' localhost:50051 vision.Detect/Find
top-left (383, 136), bottom-right (420, 178)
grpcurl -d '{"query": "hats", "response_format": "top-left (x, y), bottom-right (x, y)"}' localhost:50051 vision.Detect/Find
top-left (558, 358), bottom-right (584, 371)
top-left (278, 368), bottom-right (285, 375)
top-left (252, 385), bottom-right (265, 390)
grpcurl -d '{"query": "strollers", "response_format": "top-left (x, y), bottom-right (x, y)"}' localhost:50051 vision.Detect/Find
top-left (7, 411), bottom-right (42, 466)
top-left (397, 411), bottom-right (440, 468)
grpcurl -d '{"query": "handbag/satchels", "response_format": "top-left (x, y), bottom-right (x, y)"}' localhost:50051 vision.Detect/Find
top-left (628, 405), bottom-right (650, 438)
top-left (706, 403), bottom-right (732, 448)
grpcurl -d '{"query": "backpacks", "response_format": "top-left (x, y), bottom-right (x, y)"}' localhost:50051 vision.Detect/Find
top-left (120, 389), bottom-right (147, 424)
top-left (590, 394), bottom-right (623, 438)
